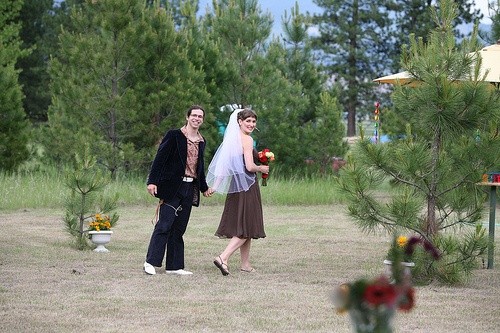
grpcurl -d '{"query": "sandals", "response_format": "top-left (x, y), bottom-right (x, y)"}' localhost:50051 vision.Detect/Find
top-left (240, 267), bottom-right (256, 274)
top-left (214, 256), bottom-right (230, 276)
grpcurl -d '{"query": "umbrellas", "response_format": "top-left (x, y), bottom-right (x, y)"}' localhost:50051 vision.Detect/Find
top-left (373, 43), bottom-right (500, 87)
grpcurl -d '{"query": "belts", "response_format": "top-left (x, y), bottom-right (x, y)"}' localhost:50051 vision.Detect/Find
top-left (183, 177), bottom-right (194, 183)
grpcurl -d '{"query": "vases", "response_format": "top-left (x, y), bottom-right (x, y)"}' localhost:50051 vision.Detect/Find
top-left (88, 231), bottom-right (114, 252)
top-left (384, 259), bottom-right (415, 280)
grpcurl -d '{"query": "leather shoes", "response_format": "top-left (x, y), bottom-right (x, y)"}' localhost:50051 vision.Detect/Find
top-left (143, 262), bottom-right (156, 275)
top-left (165, 269), bottom-right (193, 275)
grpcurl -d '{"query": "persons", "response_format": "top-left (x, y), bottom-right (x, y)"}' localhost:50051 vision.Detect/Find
top-left (205, 108), bottom-right (269, 277)
top-left (143, 105), bottom-right (212, 276)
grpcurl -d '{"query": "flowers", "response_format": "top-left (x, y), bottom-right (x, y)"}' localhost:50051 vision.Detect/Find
top-left (90, 214), bottom-right (111, 231)
top-left (387, 235), bottom-right (413, 263)
top-left (336, 278), bottom-right (414, 333)
top-left (257, 149), bottom-right (274, 186)
